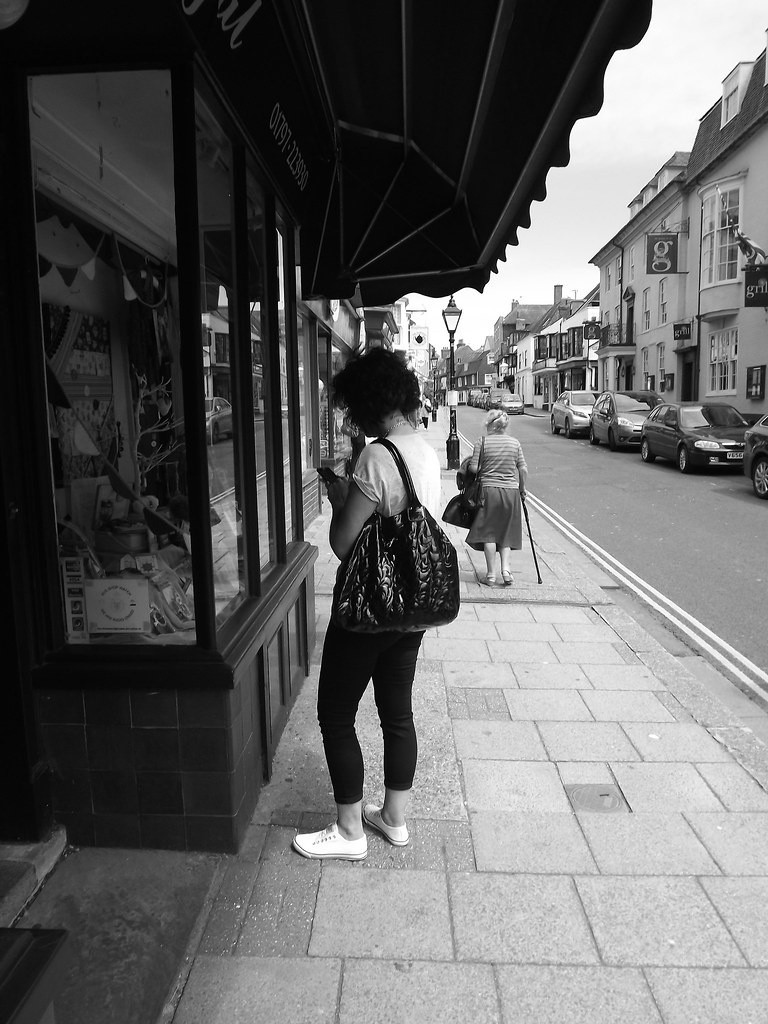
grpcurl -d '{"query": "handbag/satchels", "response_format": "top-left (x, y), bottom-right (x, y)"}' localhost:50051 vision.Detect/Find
top-left (442, 488), bottom-right (476, 529)
top-left (426, 405), bottom-right (431, 412)
top-left (464, 479), bottom-right (485, 509)
top-left (337, 438), bottom-right (461, 633)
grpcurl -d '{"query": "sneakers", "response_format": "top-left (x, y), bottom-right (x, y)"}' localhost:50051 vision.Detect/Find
top-left (293, 819), bottom-right (368, 860)
top-left (363, 804), bottom-right (409, 846)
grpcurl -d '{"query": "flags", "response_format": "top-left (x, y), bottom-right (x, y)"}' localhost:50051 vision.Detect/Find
top-left (718, 189), bottom-right (767, 266)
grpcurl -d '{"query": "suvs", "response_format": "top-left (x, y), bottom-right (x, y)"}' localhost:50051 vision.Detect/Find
top-left (487, 389), bottom-right (512, 410)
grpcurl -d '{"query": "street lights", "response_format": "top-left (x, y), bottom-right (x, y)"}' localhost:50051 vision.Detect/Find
top-left (430, 353), bottom-right (438, 422)
top-left (441, 295), bottom-right (463, 471)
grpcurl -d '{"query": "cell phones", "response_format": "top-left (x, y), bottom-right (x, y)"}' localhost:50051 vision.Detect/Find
top-left (317, 467), bottom-right (338, 485)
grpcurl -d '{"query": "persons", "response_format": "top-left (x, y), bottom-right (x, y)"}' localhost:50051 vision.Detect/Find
top-left (465, 410), bottom-right (529, 587)
top-left (421, 393), bottom-right (431, 432)
top-left (292, 348), bottom-right (442, 862)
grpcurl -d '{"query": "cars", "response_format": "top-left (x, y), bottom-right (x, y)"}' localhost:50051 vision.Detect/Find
top-left (742, 414), bottom-right (768, 500)
top-left (204, 396), bottom-right (232, 446)
top-left (467, 388), bottom-right (493, 410)
top-left (590, 390), bottom-right (666, 452)
top-left (498, 394), bottom-right (525, 415)
top-left (280, 398), bottom-right (289, 418)
top-left (639, 402), bottom-right (755, 474)
top-left (551, 390), bottom-right (604, 440)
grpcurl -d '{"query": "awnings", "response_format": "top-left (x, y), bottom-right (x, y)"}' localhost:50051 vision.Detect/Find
top-left (300, 0), bottom-right (653, 309)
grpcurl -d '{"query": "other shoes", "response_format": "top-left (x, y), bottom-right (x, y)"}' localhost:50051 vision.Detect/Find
top-left (480, 573), bottom-right (496, 586)
top-left (501, 570), bottom-right (515, 584)
